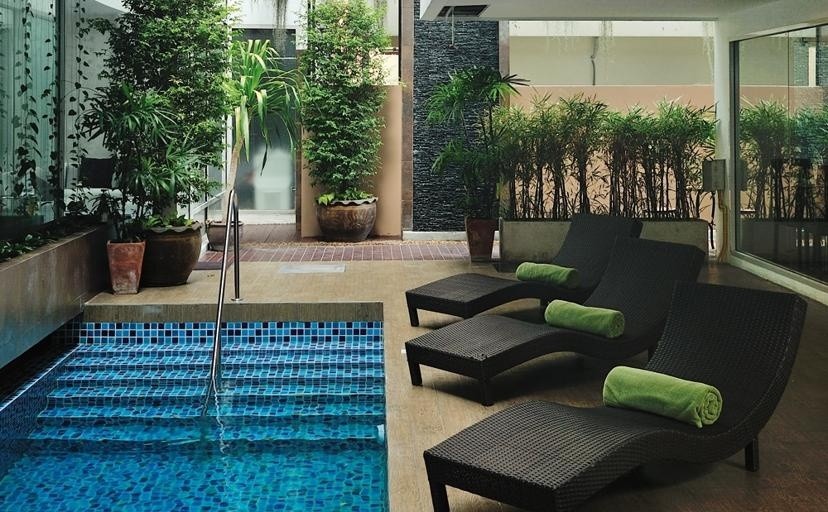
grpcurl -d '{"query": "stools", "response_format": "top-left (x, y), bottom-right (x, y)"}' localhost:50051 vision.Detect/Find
top-left (685, 186), bottom-right (716, 250)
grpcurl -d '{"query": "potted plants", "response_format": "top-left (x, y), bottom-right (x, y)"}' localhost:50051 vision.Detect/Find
top-left (422, 66), bottom-right (531, 263)
top-left (291, 0), bottom-right (407, 242)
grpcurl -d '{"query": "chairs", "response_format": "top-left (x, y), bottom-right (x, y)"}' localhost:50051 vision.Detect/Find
top-left (423, 282), bottom-right (808, 512)
top-left (406, 213), bottom-right (643, 327)
top-left (405, 234), bottom-right (706, 407)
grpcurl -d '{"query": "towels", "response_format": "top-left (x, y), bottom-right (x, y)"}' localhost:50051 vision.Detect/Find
top-left (544, 299), bottom-right (625, 338)
top-left (602, 366), bottom-right (722, 428)
top-left (516, 262), bottom-right (581, 289)
